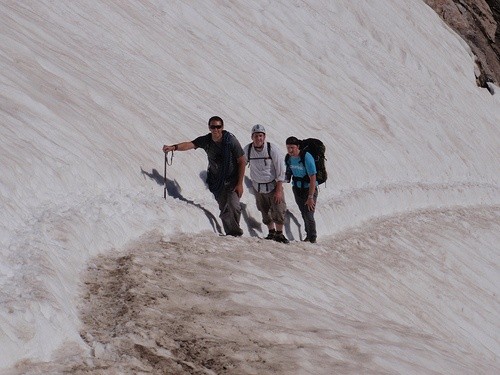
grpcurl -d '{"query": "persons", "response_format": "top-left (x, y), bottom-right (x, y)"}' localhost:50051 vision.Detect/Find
top-left (162, 116), bottom-right (245, 237)
top-left (242, 124), bottom-right (290, 244)
top-left (282, 137), bottom-right (319, 243)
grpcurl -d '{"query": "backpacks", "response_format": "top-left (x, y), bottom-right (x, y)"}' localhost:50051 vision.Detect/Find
top-left (286, 138), bottom-right (327, 184)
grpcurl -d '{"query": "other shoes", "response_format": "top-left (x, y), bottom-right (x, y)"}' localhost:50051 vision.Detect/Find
top-left (304, 237), bottom-right (316, 243)
top-left (230, 229), bottom-right (241, 236)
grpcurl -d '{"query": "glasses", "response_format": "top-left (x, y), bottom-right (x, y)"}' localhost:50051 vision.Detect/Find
top-left (210, 125), bottom-right (222, 129)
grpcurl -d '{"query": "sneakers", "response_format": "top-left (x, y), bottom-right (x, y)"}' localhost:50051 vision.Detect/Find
top-left (275, 234), bottom-right (289, 243)
top-left (265, 232), bottom-right (276, 240)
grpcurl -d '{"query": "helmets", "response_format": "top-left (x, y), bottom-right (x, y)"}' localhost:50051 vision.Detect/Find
top-left (252, 124), bottom-right (266, 136)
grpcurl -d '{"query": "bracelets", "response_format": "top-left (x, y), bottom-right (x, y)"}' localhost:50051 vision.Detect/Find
top-left (173, 145), bottom-right (178, 150)
top-left (308, 190), bottom-right (314, 195)
